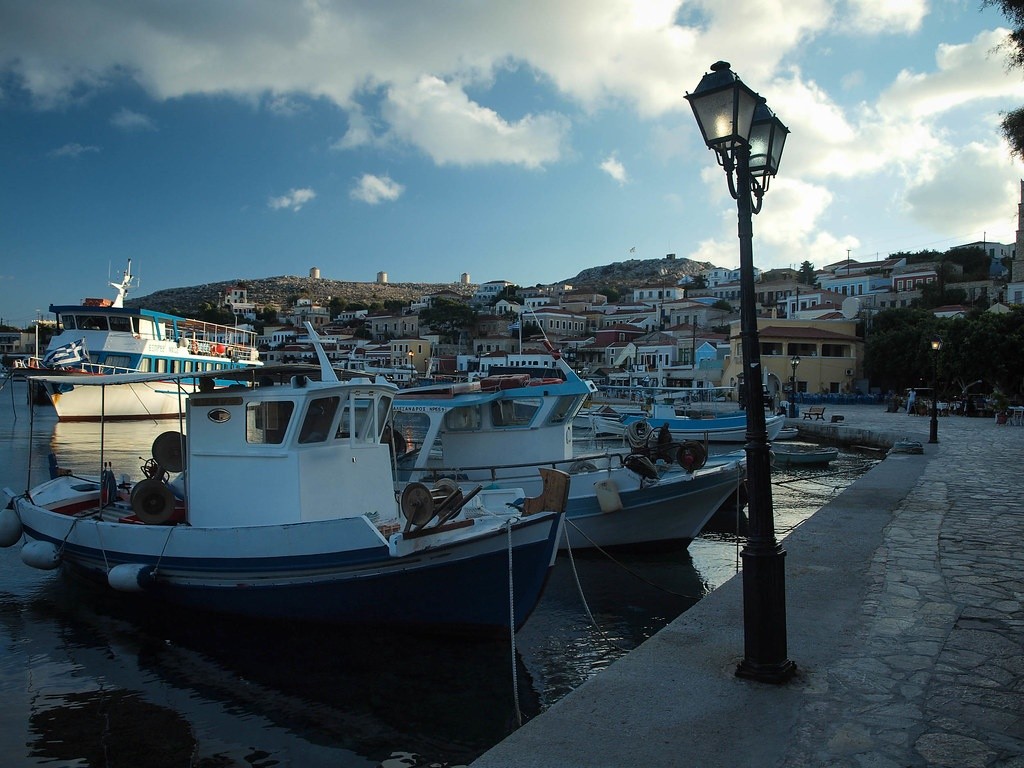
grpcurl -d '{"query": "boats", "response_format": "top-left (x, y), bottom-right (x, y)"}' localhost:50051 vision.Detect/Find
top-left (11, 320), bottom-right (572, 646)
top-left (0, 259), bottom-right (849, 560)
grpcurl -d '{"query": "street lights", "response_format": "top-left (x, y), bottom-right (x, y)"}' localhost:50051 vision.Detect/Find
top-left (785, 353), bottom-right (800, 419)
top-left (686, 58), bottom-right (800, 687)
top-left (926, 328), bottom-right (943, 445)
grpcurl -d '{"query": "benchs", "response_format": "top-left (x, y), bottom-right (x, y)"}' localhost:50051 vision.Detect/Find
top-left (801, 407), bottom-right (826, 421)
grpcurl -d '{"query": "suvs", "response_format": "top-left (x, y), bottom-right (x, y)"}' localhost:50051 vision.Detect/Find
top-left (739, 381), bottom-right (774, 410)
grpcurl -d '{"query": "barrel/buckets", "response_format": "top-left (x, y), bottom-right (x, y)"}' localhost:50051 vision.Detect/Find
top-left (593, 480), bottom-right (622, 513)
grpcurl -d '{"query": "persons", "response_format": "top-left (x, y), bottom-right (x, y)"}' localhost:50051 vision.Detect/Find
top-left (799, 391), bottom-right (803, 402)
top-left (905, 387), bottom-right (916, 416)
top-left (657, 423), bottom-right (672, 444)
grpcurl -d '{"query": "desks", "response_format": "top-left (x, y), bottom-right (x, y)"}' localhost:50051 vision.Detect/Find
top-left (1006, 406), bottom-right (1024, 425)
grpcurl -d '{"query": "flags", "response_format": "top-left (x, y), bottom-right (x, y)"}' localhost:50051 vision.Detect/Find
top-left (39, 338), bottom-right (89, 370)
top-left (507, 322), bottom-right (519, 331)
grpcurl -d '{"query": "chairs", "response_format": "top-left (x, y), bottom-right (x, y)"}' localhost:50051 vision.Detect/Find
top-left (1009, 409), bottom-right (1023, 426)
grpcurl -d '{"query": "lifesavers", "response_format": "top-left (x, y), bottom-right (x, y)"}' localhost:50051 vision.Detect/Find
top-left (211, 345), bottom-right (216, 356)
top-left (192, 342), bottom-right (199, 354)
top-left (529, 377), bottom-right (563, 386)
